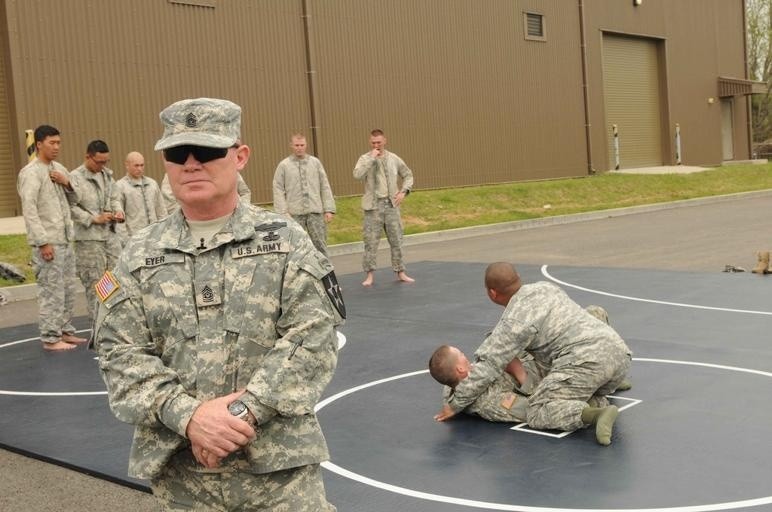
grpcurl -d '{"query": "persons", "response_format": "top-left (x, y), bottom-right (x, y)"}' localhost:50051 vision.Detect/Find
top-left (271, 133), bottom-right (336, 260)
top-left (352, 128), bottom-right (416, 287)
top-left (15, 124), bottom-right (88, 351)
top-left (68, 139), bottom-right (127, 347)
top-left (88, 95), bottom-right (348, 512)
top-left (159, 171), bottom-right (179, 216)
top-left (428, 304), bottom-right (633, 425)
top-left (433, 260), bottom-right (632, 447)
top-left (113, 151), bottom-right (170, 247)
top-left (235, 172), bottom-right (254, 204)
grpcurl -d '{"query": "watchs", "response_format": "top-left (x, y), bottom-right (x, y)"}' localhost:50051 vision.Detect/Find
top-left (228, 399), bottom-right (258, 431)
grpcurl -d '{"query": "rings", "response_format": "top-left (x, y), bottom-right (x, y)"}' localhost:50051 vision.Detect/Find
top-left (202, 449), bottom-right (208, 457)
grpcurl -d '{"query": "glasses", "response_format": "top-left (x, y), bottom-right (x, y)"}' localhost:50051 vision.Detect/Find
top-left (90, 156), bottom-right (107, 165)
top-left (163, 143), bottom-right (239, 165)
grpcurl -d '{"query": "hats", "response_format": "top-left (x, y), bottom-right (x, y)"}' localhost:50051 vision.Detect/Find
top-left (154, 97), bottom-right (242, 151)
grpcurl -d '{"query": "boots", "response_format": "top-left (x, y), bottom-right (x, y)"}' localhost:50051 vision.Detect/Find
top-left (751, 251), bottom-right (772, 274)
